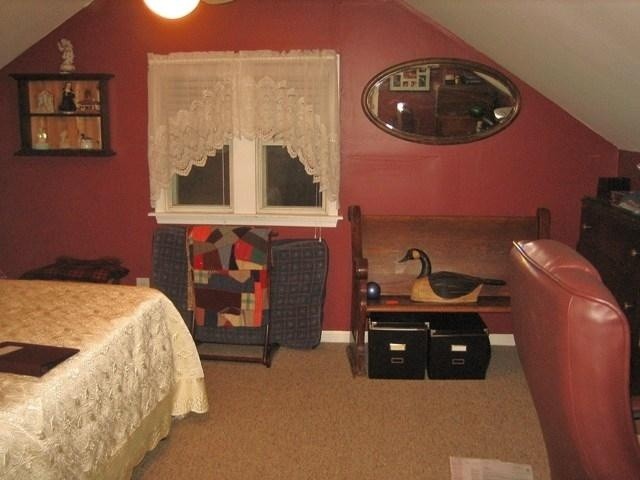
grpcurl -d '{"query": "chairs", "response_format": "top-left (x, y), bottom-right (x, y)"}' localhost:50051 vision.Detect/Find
top-left (509, 238), bottom-right (640, 480)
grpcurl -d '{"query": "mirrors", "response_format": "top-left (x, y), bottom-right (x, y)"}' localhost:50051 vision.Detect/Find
top-left (360, 56), bottom-right (523, 145)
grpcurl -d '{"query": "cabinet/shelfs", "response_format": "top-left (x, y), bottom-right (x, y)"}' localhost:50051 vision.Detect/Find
top-left (577, 198), bottom-right (640, 396)
top-left (8, 71), bottom-right (118, 159)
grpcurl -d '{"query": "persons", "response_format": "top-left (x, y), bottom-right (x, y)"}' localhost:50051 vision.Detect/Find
top-left (58, 82), bottom-right (77, 114)
top-left (56, 38), bottom-right (75, 64)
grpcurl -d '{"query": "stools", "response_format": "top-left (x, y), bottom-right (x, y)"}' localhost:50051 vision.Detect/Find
top-left (25, 265), bottom-right (129, 285)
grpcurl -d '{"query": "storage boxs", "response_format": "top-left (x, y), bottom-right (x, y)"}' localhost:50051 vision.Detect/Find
top-left (368, 313), bottom-right (491, 380)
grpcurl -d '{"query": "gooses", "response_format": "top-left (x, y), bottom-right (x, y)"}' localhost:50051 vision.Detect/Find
top-left (399, 247), bottom-right (505, 303)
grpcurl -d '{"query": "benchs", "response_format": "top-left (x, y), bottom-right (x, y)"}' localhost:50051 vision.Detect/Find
top-left (346, 205), bottom-right (551, 377)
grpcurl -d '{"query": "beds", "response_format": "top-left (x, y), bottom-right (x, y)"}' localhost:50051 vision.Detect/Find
top-left (0, 277), bottom-right (209, 480)
